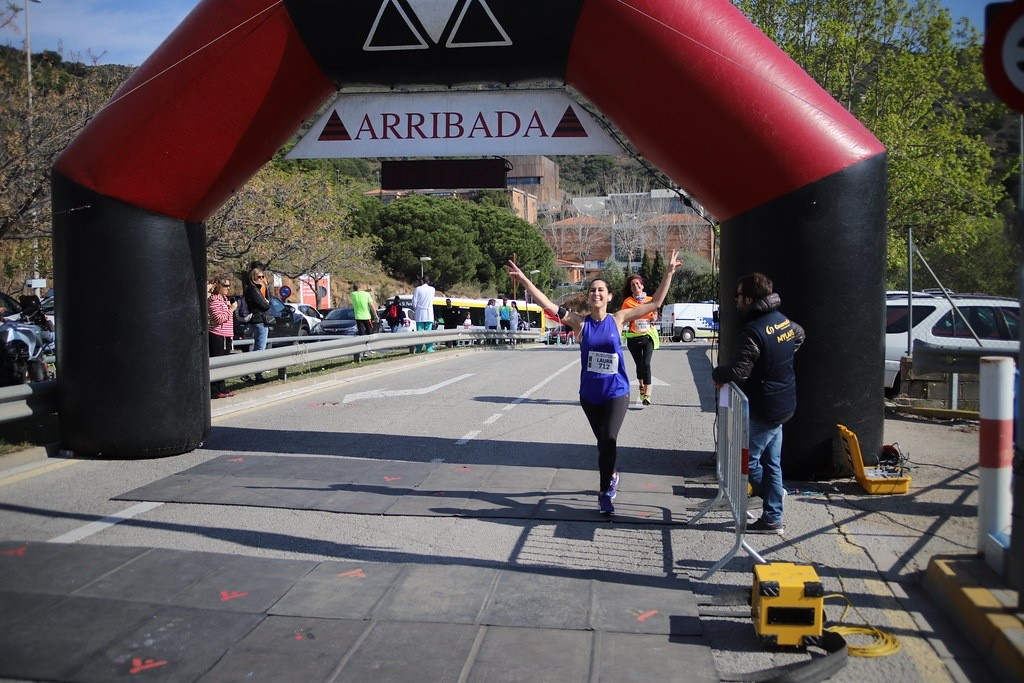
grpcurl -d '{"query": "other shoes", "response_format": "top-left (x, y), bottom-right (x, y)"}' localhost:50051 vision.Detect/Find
top-left (254, 378), bottom-right (270, 383)
top-left (222, 390), bottom-right (236, 396)
top-left (363, 352), bottom-right (368, 357)
top-left (417, 347), bottom-right (423, 353)
top-left (213, 393), bottom-right (229, 398)
top-left (426, 347), bottom-right (435, 353)
top-left (370, 350), bottom-right (376, 354)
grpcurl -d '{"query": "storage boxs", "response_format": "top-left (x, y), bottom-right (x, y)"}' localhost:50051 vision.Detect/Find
top-left (837, 425), bottom-right (911, 494)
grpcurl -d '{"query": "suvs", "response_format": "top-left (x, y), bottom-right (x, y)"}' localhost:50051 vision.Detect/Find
top-left (884, 294), bottom-right (1020, 401)
top-left (547, 325), bottom-right (580, 344)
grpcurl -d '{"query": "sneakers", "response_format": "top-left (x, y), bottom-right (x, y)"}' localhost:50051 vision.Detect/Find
top-left (640, 386), bottom-right (644, 401)
top-left (606, 473), bottom-right (619, 499)
top-left (782, 488), bottom-right (787, 500)
top-left (745, 518), bottom-right (783, 534)
top-left (642, 395), bottom-right (651, 405)
top-left (598, 494), bottom-right (614, 514)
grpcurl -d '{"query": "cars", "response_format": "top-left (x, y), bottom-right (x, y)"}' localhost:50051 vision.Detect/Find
top-left (318, 308), bottom-right (332, 317)
top-left (622, 320), bottom-right (661, 334)
top-left (284, 303), bottom-right (324, 336)
top-left (0, 289), bottom-right (55, 326)
top-left (377, 308), bottom-right (417, 332)
top-left (317, 307), bottom-right (383, 341)
top-left (229, 294), bottom-right (311, 351)
top-left (556, 280), bottom-right (593, 293)
top-left (538, 327), bottom-right (550, 341)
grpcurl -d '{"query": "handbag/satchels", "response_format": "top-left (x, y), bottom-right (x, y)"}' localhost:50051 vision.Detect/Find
top-left (263, 315), bottom-right (277, 325)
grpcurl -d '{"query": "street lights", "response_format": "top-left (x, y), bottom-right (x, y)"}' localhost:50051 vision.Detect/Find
top-left (530, 270), bottom-right (540, 304)
top-left (421, 257), bottom-right (431, 277)
top-left (25, 0), bottom-right (41, 298)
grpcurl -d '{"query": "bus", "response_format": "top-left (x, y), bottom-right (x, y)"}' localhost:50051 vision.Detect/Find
top-left (385, 295), bottom-right (544, 342)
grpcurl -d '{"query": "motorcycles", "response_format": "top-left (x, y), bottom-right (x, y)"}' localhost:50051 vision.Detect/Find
top-left (0, 295), bottom-right (57, 385)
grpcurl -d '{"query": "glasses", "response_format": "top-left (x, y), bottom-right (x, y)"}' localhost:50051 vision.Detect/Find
top-left (219, 281), bottom-right (230, 288)
top-left (735, 291), bottom-right (746, 297)
top-left (257, 275), bottom-right (264, 278)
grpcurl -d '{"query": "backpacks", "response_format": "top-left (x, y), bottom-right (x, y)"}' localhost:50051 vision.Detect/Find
top-left (388, 306), bottom-right (397, 318)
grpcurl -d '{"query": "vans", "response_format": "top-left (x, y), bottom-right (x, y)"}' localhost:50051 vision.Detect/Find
top-left (661, 300), bottom-right (720, 342)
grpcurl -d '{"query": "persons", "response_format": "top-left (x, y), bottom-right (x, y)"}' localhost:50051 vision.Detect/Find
top-left (484, 298), bottom-right (500, 346)
top-left (381, 295), bottom-right (405, 331)
top-left (244, 268), bottom-right (271, 383)
top-left (206, 273), bottom-right (239, 398)
top-left (499, 297), bottom-right (512, 344)
top-left (441, 299), bottom-right (460, 348)
top-left (350, 283), bottom-right (380, 360)
top-left (503, 248), bottom-right (683, 513)
top-left (18, 295), bottom-right (53, 381)
top-left (509, 301), bottom-right (519, 344)
top-left (463, 312), bottom-right (472, 329)
top-left (619, 275), bottom-right (660, 406)
top-left (411, 275), bottom-right (436, 353)
top-left (712, 273), bottom-right (806, 535)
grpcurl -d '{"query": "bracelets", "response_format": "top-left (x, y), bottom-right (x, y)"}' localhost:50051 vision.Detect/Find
top-left (374, 316), bottom-right (378, 319)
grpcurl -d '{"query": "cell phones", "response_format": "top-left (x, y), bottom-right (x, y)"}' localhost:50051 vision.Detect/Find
top-left (230, 297), bottom-right (235, 305)
